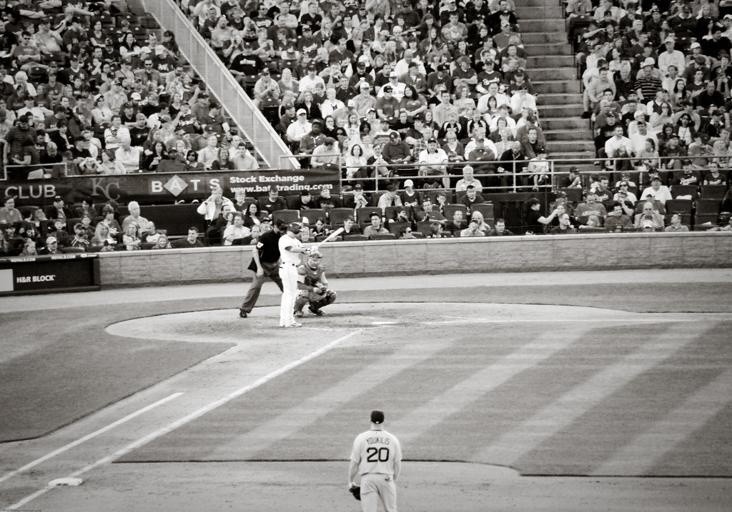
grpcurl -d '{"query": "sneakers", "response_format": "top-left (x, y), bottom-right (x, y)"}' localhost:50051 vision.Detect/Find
top-left (239, 309), bottom-right (326, 328)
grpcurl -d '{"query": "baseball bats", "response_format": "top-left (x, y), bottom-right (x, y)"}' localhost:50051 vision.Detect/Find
top-left (317, 227), bottom-right (345, 248)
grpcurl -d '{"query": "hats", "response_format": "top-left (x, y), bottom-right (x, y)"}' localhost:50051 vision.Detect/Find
top-left (238, 2), bottom-right (514, 129)
top-left (605, 7), bottom-right (732, 121)
top-left (371, 410), bottom-right (385, 424)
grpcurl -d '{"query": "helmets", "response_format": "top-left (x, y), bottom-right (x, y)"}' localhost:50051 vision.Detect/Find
top-left (287, 222), bottom-right (304, 235)
top-left (307, 251), bottom-right (323, 270)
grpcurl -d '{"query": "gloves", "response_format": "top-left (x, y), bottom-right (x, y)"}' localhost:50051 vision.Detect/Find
top-left (350, 486), bottom-right (360, 500)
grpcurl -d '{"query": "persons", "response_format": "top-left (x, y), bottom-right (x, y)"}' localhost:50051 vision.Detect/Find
top-left (1, 1), bottom-right (732, 258)
top-left (278, 222), bottom-right (306, 328)
top-left (239, 220), bottom-right (286, 318)
top-left (347, 409), bottom-right (402, 512)
top-left (292, 248), bottom-right (336, 316)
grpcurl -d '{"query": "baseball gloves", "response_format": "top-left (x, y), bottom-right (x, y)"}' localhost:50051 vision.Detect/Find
top-left (349, 485), bottom-right (359, 500)
top-left (317, 286), bottom-right (327, 295)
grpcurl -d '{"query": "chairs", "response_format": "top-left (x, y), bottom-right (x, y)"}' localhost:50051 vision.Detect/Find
top-left (1, 171), bottom-right (732, 252)
